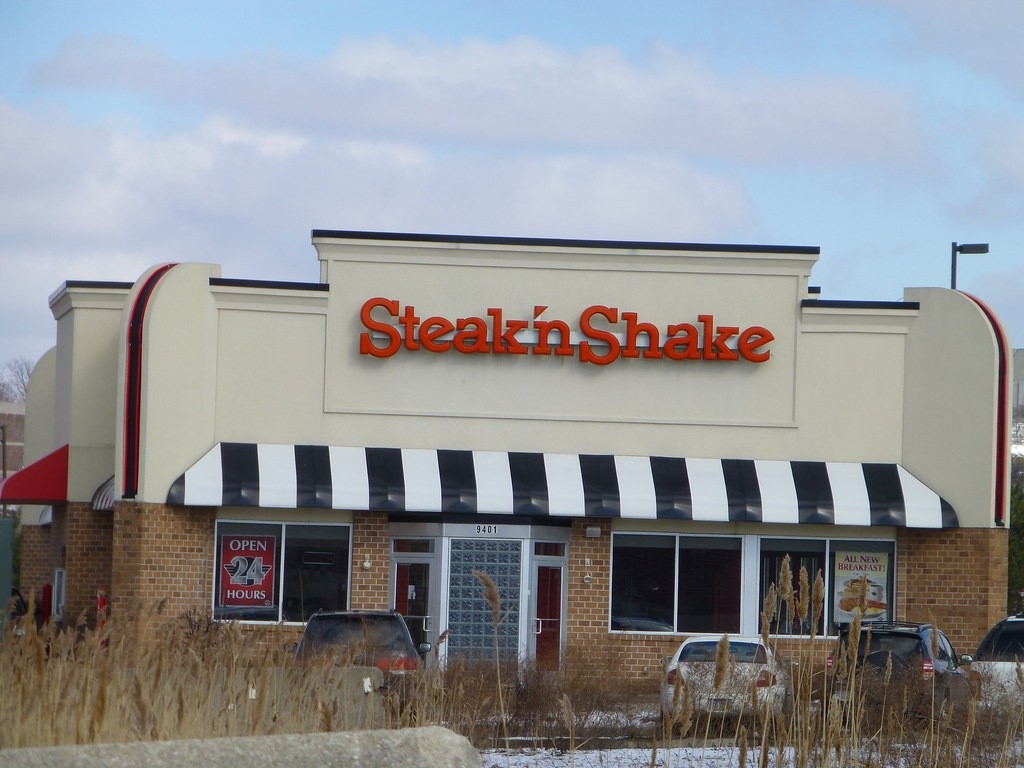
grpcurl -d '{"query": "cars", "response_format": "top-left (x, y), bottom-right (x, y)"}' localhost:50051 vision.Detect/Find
top-left (660, 633), bottom-right (790, 737)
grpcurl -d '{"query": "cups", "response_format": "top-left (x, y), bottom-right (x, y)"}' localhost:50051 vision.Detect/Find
top-left (869, 584), bottom-right (884, 602)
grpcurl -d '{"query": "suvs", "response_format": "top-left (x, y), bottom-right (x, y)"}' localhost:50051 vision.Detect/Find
top-left (287, 608), bottom-right (433, 729)
top-left (963, 611), bottom-right (1024, 737)
top-left (817, 620), bottom-right (975, 734)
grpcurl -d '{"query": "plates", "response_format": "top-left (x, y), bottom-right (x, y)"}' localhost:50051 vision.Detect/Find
top-left (838, 603), bottom-right (887, 618)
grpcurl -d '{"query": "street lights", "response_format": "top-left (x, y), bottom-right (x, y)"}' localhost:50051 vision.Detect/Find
top-left (950, 241), bottom-right (991, 291)
